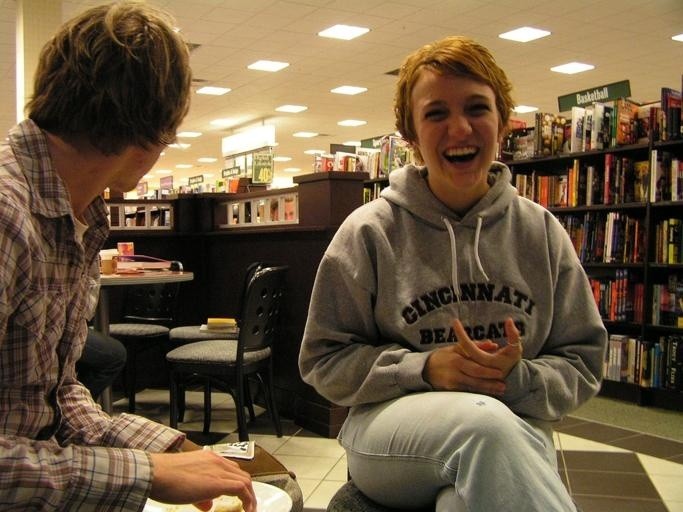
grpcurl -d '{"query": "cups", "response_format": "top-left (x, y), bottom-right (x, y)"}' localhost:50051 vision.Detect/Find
top-left (117, 241), bottom-right (134, 256)
top-left (101, 260), bottom-right (118, 274)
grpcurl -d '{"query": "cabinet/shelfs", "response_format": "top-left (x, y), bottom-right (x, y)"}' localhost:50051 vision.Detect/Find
top-left (506, 86), bottom-right (682, 412)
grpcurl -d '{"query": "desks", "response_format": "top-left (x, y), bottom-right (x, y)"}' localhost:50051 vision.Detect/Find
top-left (96, 271), bottom-right (194, 415)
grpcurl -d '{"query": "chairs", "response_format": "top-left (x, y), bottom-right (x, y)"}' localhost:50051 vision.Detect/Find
top-left (168, 262), bottom-right (277, 422)
top-left (165, 266), bottom-right (289, 442)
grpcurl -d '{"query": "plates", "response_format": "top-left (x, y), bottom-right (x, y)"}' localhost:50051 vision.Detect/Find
top-left (141, 479), bottom-right (293, 512)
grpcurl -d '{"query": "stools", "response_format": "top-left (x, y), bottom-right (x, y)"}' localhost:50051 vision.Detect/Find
top-left (326, 468), bottom-right (388, 511)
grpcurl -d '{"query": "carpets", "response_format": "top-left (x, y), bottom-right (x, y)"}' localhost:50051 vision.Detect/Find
top-left (564, 393), bottom-right (683, 444)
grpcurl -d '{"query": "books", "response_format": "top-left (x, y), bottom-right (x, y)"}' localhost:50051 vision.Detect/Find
top-left (100, 180), bottom-right (299, 276)
top-left (199, 318), bottom-right (239, 334)
top-left (494, 87), bottom-right (683, 391)
top-left (317, 136), bottom-right (416, 204)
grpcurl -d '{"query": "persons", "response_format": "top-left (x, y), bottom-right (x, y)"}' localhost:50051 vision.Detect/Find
top-left (298, 35), bottom-right (609, 512)
top-left (0, 1), bottom-right (260, 512)
